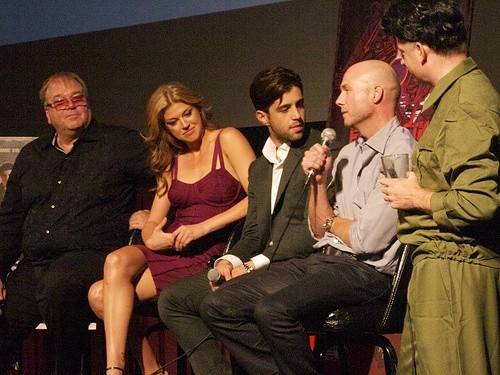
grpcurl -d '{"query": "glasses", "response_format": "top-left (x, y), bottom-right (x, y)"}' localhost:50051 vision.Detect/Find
top-left (44, 95), bottom-right (88, 110)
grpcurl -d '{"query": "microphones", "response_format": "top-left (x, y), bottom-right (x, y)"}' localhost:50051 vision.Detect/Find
top-left (305, 127), bottom-right (336, 185)
top-left (208, 269), bottom-right (226, 286)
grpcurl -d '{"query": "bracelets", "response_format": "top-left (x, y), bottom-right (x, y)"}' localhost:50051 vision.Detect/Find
top-left (242, 262), bottom-right (254, 273)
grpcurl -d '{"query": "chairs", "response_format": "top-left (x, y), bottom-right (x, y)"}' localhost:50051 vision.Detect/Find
top-left (312, 244), bottom-right (417, 375)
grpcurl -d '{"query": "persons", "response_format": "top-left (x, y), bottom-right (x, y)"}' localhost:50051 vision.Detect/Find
top-left (0, 72), bottom-right (155, 375)
top-left (158, 66), bottom-right (343, 375)
top-left (200, 60), bottom-right (418, 375)
top-left (88, 82), bottom-right (257, 375)
top-left (378, 0), bottom-right (500, 375)
top-left (0, 162), bottom-right (13, 203)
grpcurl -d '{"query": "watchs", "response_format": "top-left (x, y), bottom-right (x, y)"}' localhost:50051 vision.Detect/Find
top-left (324, 215), bottom-right (337, 232)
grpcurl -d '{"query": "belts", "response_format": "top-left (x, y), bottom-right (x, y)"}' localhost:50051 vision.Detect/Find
top-left (317, 244), bottom-right (362, 261)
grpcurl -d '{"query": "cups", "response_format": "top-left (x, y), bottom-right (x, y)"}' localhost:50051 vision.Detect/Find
top-left (380, 154), bottom-right (410, 179)
